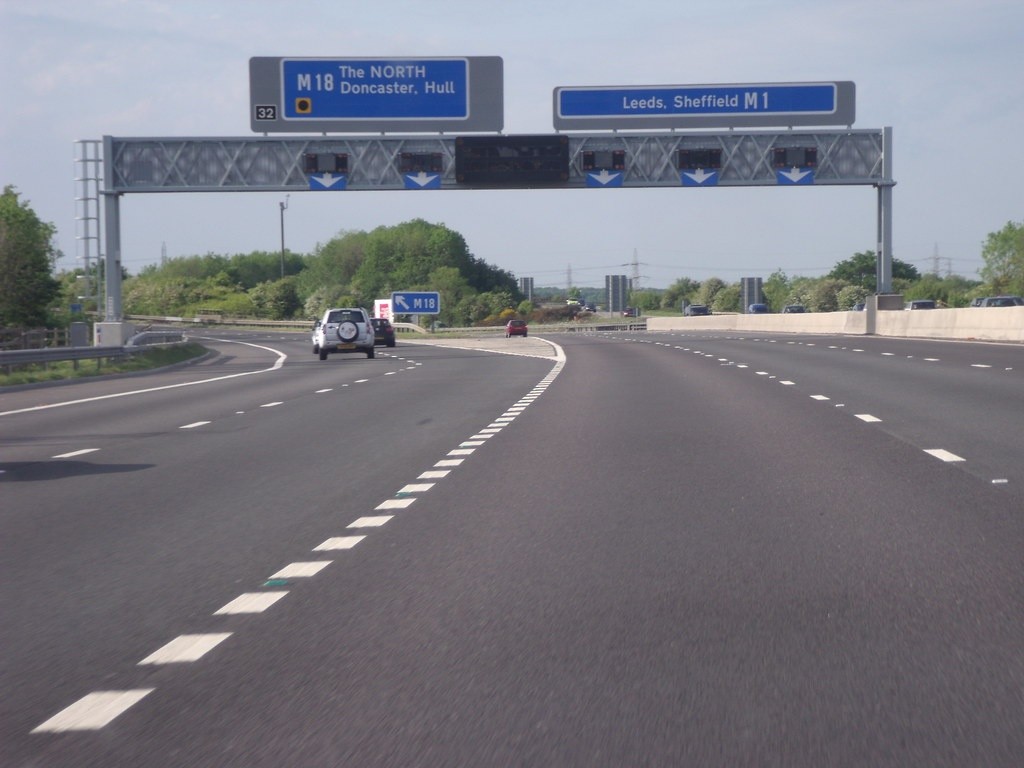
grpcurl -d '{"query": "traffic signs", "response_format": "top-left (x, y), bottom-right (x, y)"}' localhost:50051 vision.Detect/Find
top-left (391, 291), bottom-right (440, 315)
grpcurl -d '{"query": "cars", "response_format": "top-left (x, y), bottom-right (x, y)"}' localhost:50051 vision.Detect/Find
top-left (585, 303), bottom-right (596, 313)
top-left (567, 298), bottom-right (580, 307)
top-left (370, 318), bottom-right (396, 347)
top-left (312, 318), bottom-right (323, 355)
top-left (747, 303), bottom-right (767, 313)
top-left (506, 319), bottom-right (529, 338)
top-left (684, 303), bottom-right (710, 316)
top-left (852, 302), bottom-right (866, 311)
top-left (621, 307), bottom-right (637, 318)
top-left (908, 299), bottom-right (936, 310)
top-left (969, 297), bottom-right (985, 307)
top-left (979, 296), bottom-right (1016, 308)
top-left (1012, 297), bottom-right (1024, 305)
top-left (782, 305), bottom-right (806, 314)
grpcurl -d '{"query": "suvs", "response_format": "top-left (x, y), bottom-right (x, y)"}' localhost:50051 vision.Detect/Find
top-left (314, 306), bottom-right (375, 360)
